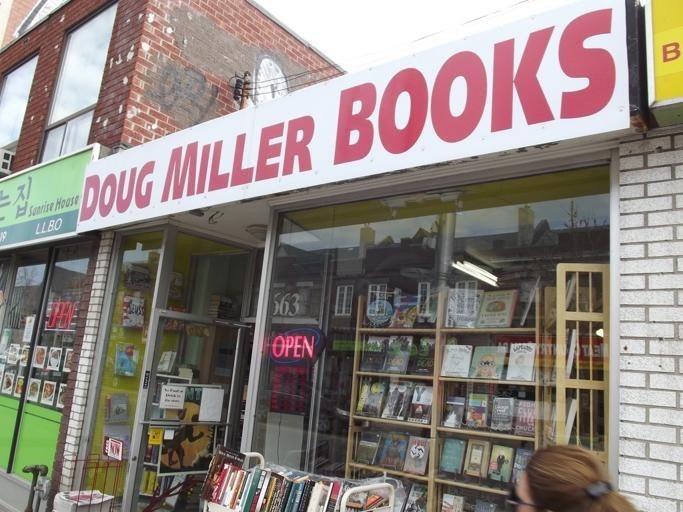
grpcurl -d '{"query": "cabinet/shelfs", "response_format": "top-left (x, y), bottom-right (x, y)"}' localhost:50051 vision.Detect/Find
top-left (342, 266), bottom-right (604, 512)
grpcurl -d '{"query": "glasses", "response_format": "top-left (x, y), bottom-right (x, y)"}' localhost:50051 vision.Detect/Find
top-left (504, 496), bottom-right (539, 512)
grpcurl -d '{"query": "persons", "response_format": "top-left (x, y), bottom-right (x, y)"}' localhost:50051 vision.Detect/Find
top-left (506, 445), bottom-right (637, 511)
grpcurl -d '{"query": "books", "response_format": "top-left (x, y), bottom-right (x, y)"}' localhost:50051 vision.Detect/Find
top-left (101, 259), bottom-right (235, 498)
top-left (52, 489), bottom-right (114, 512)
top-left (353, 260), bottom-right (578, 512)
top-left (199, 445), bottom-right (391, 512)
